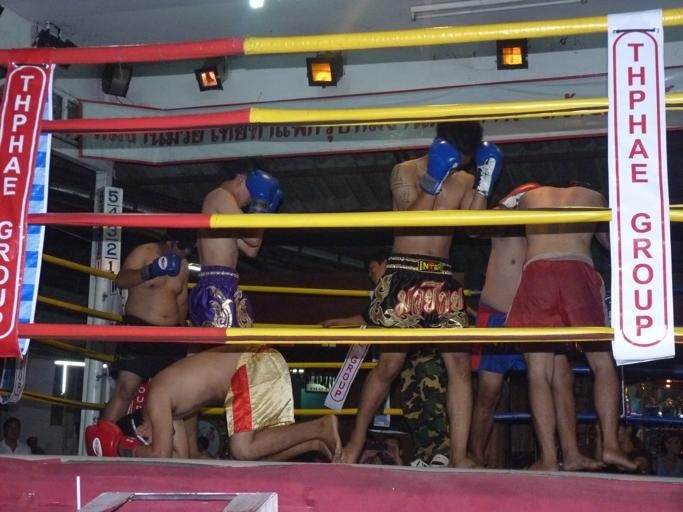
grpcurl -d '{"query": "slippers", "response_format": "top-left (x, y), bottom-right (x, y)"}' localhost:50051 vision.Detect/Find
top-left (429, 454), bottom-right (449, 467)
top-left (409, 458), bottom-right (429, 467)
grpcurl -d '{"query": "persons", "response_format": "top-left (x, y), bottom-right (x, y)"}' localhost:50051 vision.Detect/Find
top-left (84, 342), bottom-right (341, 465)
top-left (511, 185), bottom-right (644, 472)
top-left (192, 159), bottom-right (281, 350)
top-left (362, 434), bottom-right (414, 466)
top-left (342, 117), bottom-right (486, 470)
top-left (465, 182), bottom-right (609, 472)
top-left (0, 405), bottom-right (80, 456)
top-left (617, 421), bottom-right (682, 478)
top-left (321, 251), bottom-right (453, 468)
top-left (98, 226), bottom-right (209, 458)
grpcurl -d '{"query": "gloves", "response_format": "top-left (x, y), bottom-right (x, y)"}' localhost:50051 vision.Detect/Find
top-left (245, 168), bottom-right (283, 213)
top-left (85, 420), bottom-right (142, 458)
top-left (471, 140), bottom-right (504, 199)
top-left (141, 253), bottom-right (180, 281)
top-left (498, 181), bottom-right (541, 208)
top-left (418, 138), bottom-right (462, 196)
